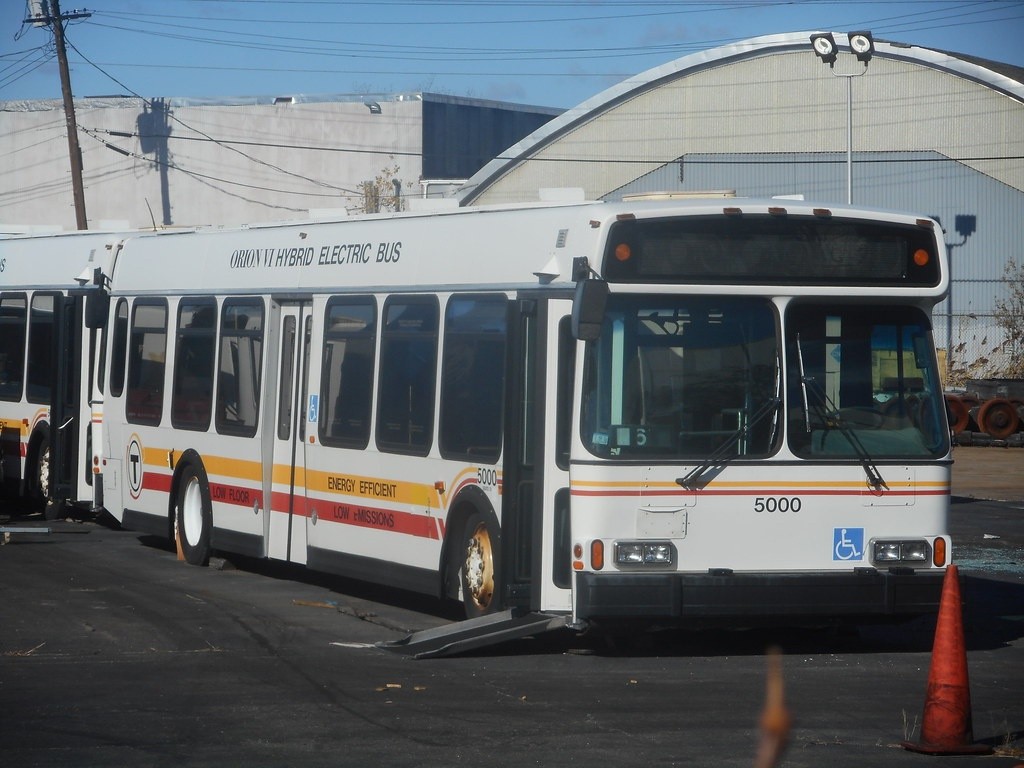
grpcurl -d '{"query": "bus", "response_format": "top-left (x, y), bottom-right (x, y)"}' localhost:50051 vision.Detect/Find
top-left (0, 191), bottom-right (953, 634)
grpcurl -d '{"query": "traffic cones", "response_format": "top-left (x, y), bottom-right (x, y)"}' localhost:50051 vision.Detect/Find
top-left (900, 564), bottom-right (992, 757)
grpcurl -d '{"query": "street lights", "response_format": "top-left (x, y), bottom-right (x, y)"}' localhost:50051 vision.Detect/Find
top-left (810, 30), bottom-right (875, 206)
